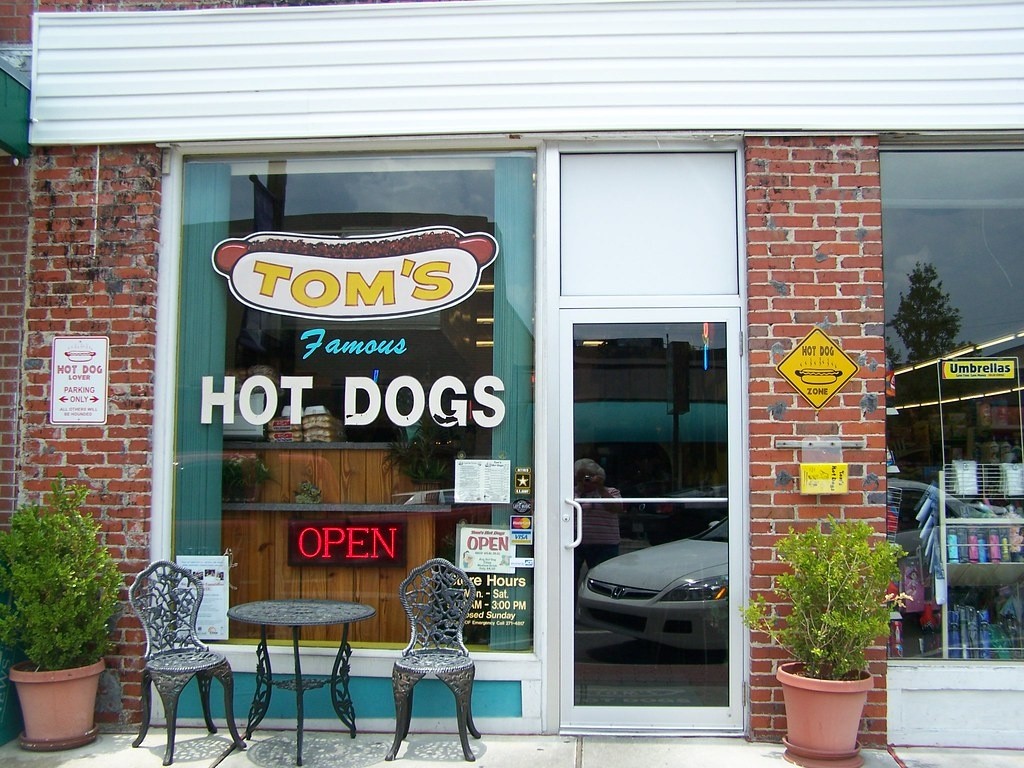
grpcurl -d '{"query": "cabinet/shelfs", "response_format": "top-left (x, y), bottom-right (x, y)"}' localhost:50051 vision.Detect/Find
top-left (223, 443), bottom-right (453, 644)
top-left (938, 425), bottom-right (1024, 662)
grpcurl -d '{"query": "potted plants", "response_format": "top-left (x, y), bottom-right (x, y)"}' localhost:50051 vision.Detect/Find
top-left (294, 481), bottom-right (323, 504)
top-left (0, 470), bottom-right (125, 751)
top-left (236, 456), bottom-right (270, 502)
top-left (739, 512), bottom-right (910, 768)
top-left (382, 415), bottom-right (458, 505)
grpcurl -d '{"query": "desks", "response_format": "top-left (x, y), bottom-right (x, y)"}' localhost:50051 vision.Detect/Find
top-left (227, 601), bottom-right (377, 766)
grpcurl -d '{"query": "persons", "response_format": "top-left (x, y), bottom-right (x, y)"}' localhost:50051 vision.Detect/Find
top-left (574, 458), bottom-right (623, 585)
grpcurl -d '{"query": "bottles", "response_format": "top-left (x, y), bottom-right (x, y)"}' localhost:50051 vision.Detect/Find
top-left (899, 398), bottom-right (975, 449)
top-left (942, 379), bottom-right (970, 384)
top-left (976, 392), bottom-right (1024, 426)
top-left (976, 430), bottom-right (1024, 463)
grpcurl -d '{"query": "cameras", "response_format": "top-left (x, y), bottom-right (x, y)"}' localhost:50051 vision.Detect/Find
top-left (584, 476), bottom-right (591, 482)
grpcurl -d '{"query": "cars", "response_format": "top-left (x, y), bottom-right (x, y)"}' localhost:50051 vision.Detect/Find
top-left (617, 484), bottom-right (728, 546)
top-left (575, 477), bottom-right (1023, 652)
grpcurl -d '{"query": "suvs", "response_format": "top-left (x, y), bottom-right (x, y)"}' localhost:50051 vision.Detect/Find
top-left (390, 487), bottom-right (505, 541)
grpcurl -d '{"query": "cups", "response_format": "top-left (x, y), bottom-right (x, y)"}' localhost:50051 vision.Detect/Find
top-left (948, 527), bottom-right (1011, 564)
top-left (948, 606), bottom-right (991, 659)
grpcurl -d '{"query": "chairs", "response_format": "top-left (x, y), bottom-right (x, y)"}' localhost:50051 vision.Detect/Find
top-left (129, 560), bottom-right (247, 767)
top-left (385, 558), bottom-right (481, 762)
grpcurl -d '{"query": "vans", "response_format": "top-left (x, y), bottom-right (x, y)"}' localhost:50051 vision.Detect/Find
top-left (175, 451), bottom-right (357, 592)
